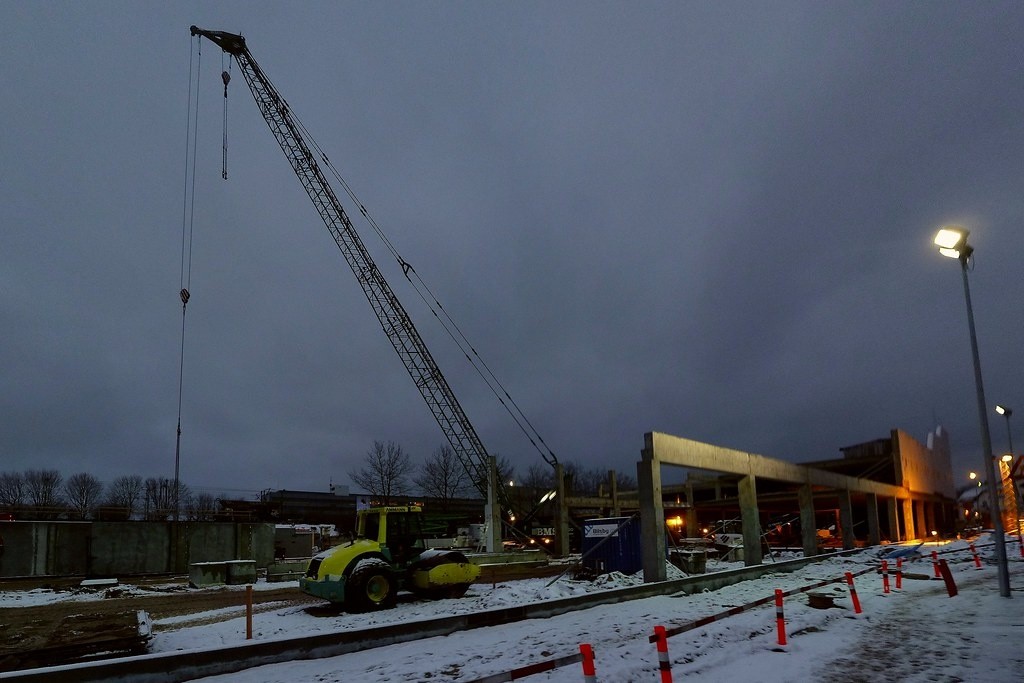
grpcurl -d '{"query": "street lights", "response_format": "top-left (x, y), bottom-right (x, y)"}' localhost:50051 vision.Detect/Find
top-left (933, 223), bottom-right (1011, 600)
top-left (995, 404), bottom-right (1013, 453)
top-left (969, 472), bottom-right (981, 534)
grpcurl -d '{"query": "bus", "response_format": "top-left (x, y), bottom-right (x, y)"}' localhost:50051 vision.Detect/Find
top-left (581, 515), bottom-right (635, 539)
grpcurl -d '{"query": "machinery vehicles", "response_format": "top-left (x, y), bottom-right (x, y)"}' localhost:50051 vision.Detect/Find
top-left (164, 23), bottom-right (602, 554)
top-left (298, 501), bottom-right (482, 613)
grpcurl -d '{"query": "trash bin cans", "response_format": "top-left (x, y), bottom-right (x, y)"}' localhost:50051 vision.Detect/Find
top-left (227, 560), bottom-right (259, 585)
top-left (188, 561), bottom-right (227, 585)
